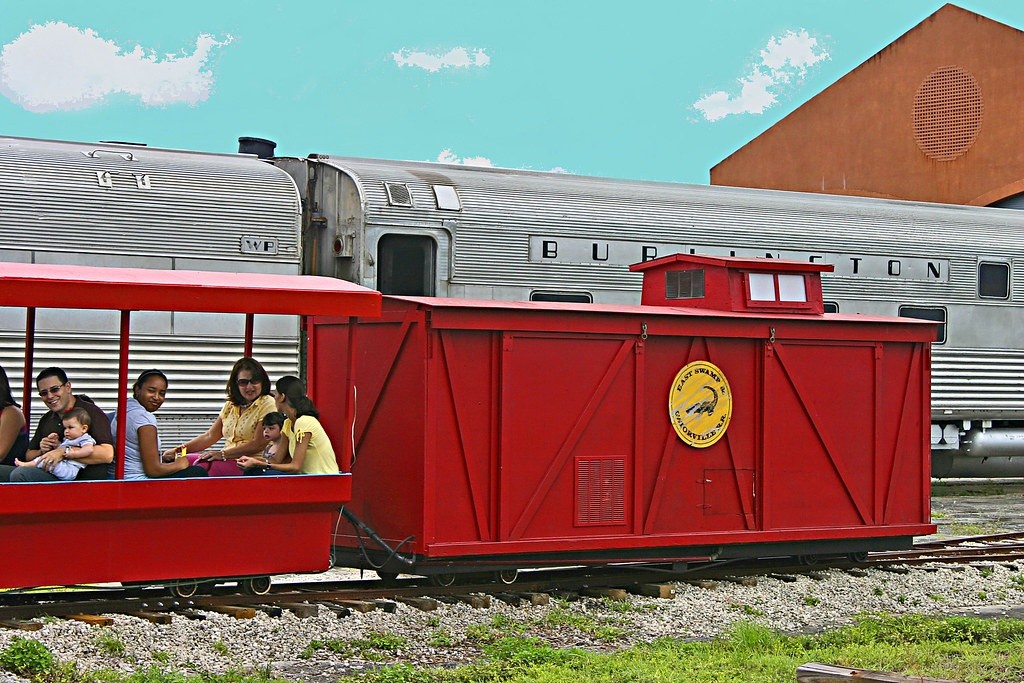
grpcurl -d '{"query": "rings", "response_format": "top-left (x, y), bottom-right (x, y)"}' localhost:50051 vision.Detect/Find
top-left (48, 460), bottom-right (52, 463)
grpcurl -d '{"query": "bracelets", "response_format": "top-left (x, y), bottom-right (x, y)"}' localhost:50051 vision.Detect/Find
top-left (266, 463), bottom-right (271, 470)
top-left (221, 450), bottom-right (226, 461)
top-left (65, 446), bottom-right (69, 453)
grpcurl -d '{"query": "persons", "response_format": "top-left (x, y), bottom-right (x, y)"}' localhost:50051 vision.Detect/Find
top-left (0, 367), bottom-right (114, 482)
top-left (236, 376), bottom-right (339, 477)
top-left (0, 366), bottom-right (29, 466)
top-left (163, 357), bottom-right (278, 476)
top-left (107, 369), bottom-right (209, 480)
top-left (14, 407), bottom-right (96, 481)
top-left (262, 412), bottom-right (287, 458)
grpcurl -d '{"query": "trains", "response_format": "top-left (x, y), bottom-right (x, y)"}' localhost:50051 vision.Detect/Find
top-left (0, 135), bottom-right (1023, 483)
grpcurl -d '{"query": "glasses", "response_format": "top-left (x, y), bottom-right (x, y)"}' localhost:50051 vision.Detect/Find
top-left (235, 375), bottom-right (262, 387)
top-left (38, 380), bottom-right (67, 396)
